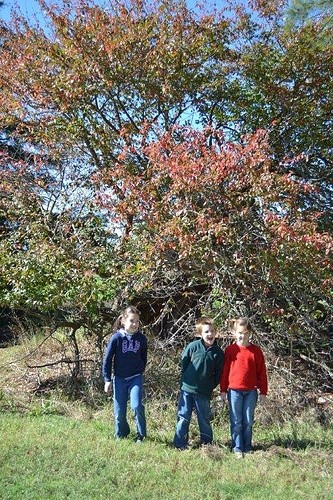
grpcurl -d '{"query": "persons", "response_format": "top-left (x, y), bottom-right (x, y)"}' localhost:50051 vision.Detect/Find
top-left (173, 317), bottom-right (225, 448)
top-left (103, 306), bottom-right (148, 443)
top-left (219, 317), bottom-right (269, 459)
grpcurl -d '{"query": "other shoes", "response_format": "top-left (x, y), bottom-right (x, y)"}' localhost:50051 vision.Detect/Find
top-left (115, 438), bottom-right (121, 442)
top-left (236, 452), bottom-right (243, 459)
top-left (137, 440), bottom-right (142, 443)
